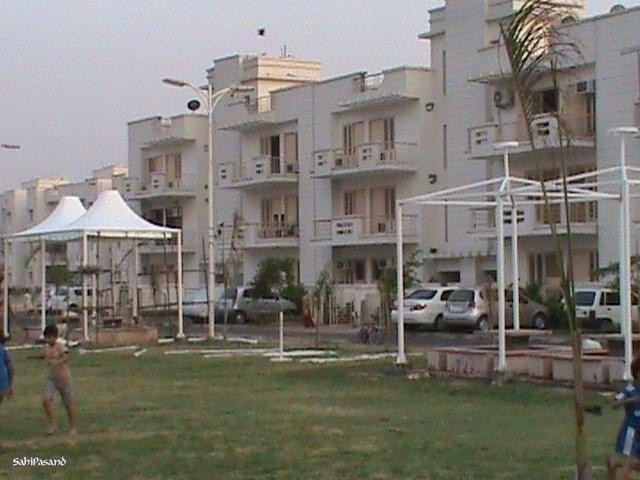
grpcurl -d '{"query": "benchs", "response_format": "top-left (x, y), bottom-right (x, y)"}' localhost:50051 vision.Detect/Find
top-left (24, 323), bottom-right (160, 347)
top-left (429, 343), bottom-right (635, 383)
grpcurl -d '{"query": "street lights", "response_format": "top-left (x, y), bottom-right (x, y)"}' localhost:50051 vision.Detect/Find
top-left (0, 144), bottom-right (20, 150)
top-left (162, 76), bottom-right (257, 340)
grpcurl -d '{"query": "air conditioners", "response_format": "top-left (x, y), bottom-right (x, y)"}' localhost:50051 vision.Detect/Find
top-left (493, 88), bottom-right (514, 108)
top-left (574, 79), bottom-right (594, 96)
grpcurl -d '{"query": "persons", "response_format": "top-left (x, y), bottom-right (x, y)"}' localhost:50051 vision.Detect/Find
top-left (605, 355), bottom-right (639, 480)
top-left (42, 324), bottom-right (79, 435)
top-left (0, 341), bottom-right (16, 404)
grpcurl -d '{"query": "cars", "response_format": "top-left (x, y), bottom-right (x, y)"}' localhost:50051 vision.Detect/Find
top-left (388, 287), bottom-right (640, 335)
top-left (32, 284), bottom-right (102, 314)
top-left (184, 285), bottom-right (299, 324)
